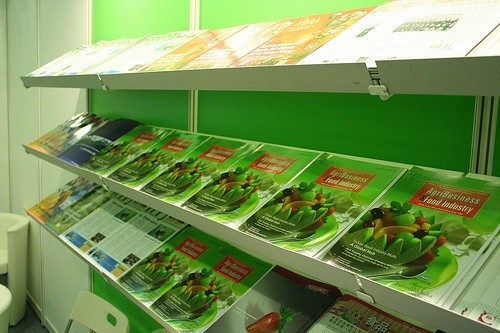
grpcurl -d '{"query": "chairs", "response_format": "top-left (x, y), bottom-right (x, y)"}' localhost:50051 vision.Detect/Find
top-left (0, 213), bottom-right (29, 326)
top-left (64, 290), bottom-right (128, 333)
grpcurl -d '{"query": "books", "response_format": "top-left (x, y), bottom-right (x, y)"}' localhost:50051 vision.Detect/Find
top-left (56, 117), bottom-right (142, 167)
top-left (317, 158), bottom-right (500, 307)
top-left (28, 112), bottom-right (110, 156)
top-left (88, 28), bottom-right (208, 72)
top-left (79, 123), bottom-right (176, 178)
top-left (139, 134), bottom-right (263, 207)
top-left (236, 150), bottom-right (407, 262)
top-left (227, 2), bottom-right (377, 67)
top-left (45, 185), bottom-right (113, 235)
top-left (202, 263), bottom-right (342, 333)
top-left (181, 143), bottom-right (321, 227)
top-left (137, 23), bottom-right (246, 73)
top-left (59, 192), bottom-right (150, 255)
top-left (441, 230), bottom-right (500, 311)
top-left (35, 30), bottom-right (150, 75)
top-left (28, 174), bottom-right (101, 224)
top-left (83, 206), bottom-right (185, 278)
top-left (114, 225), bottom-right (228, 305)
top-left (147, 243), bottom-right (276, 333)
top-left (179, 19), bottom-right (301, 68)
top-left (307, 293), bottom-right (433, 333)
top-left (24, 44), bottom-right (88, 77)
top-left (296, 0), bottom-right (500, 63)
top-left (104, 130), bottom-right (213, 191)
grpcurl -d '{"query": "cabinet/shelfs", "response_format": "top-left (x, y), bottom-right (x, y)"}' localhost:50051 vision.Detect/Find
top-left (19, 0), bottom-right (499, 333)
top-left (91, 1), bottom-right (482, 332)
